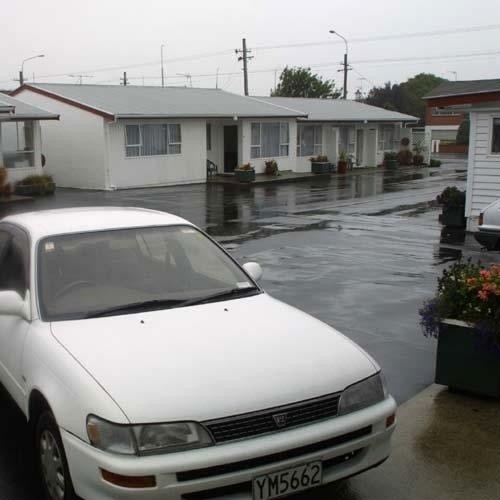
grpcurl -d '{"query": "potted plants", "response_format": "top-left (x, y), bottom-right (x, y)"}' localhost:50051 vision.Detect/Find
top-left (308, 155), bottom-right (333, 173)
top-left (265, 160), bottom-right (278, 175)
top-left (436, 187), bottom-right (466, 227)
top-left (337, 148), bottom-right (349, 173)
top-left (16, 173), bottom-right (55, 194)
top-left (383, 151), bottom-right (399, 169)
top-left (234, 162), bottom-right (255, 183)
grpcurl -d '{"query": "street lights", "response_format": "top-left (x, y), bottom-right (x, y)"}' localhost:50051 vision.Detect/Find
top-left (330, 30), bottom-right (348, 98)
top-left (20, 54), bottom-right (45, 85)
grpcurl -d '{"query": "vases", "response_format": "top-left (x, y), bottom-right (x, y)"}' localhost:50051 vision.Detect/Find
top-left (433, 315), bottom-right (499, 403)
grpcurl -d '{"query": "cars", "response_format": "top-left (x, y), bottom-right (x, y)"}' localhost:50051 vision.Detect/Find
top-left (0, 206), bottom-right (397, 499)
top-left (473, 199), bottom-right (500, 251)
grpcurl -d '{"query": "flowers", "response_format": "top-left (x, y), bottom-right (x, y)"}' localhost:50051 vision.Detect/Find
top-left (416, 255), bottom-right (500, 347)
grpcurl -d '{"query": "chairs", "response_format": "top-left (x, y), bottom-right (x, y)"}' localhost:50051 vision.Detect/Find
top-left (44, 240), bottom-right (158, 297)
top-left (207, 159), bottom-right (218, 180)
